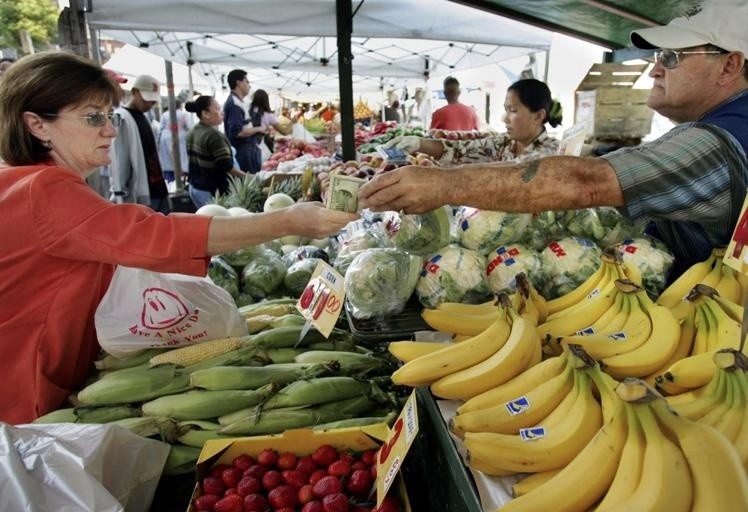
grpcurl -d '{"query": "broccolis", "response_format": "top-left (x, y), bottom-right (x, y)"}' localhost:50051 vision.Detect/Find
top-left (344, 248), bottom-right (421, 318)
top-left (415, 246), bottom-right (493, 309)
top-left (334, 206), bottom-right (638, 273)
top-left (617, 236), bottom-right (674, 292)
top-left (486, 245), bottom-right (540, 295)
top-left (541, 238), bottom-right (603, 300)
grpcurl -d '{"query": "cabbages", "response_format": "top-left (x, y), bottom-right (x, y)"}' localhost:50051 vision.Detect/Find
top-left (207, 242), bottom-right (329, 308)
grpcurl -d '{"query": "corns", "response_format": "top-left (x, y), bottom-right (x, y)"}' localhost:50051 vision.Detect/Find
top-left (30, 299), bottom-right (399, 476)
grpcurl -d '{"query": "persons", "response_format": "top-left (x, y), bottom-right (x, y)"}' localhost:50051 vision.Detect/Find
top-left (185, 96), bottom-right (246, 210)
top-left (408, 88), bottom-right (424, 121)
top-left (157, 100), bottom-right (190, 192)
top-left (429, 76), bottom-right (478, 131)
top-left (288, 101), bottom-right (329, 123)
top-left (382, 78), bottom-right (567, 164)
top-left (222, 69), bottom-right (266, 174)
top-left (385, 101), bottom-right (400, 123)
top-left (272, 107), bottom-right (290, 153)
top-left (357, 1), bottom-right (748, 265)
top-left (108, 74), bottom-right (172, 218)
top-left (0, 50), bottom-right (362, 426)
top-left (247, 89), bottom-right (304, 167)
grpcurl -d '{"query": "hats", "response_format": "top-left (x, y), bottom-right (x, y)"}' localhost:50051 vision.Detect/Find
top-left (106, 69), bottom-right (129, 83)
top-left (629, 0), bottom-right (747, 59)
top-left (133, 74), bottom-right (162, 105)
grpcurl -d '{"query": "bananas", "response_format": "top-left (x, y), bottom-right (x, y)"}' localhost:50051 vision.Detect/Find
top-left (664, 348), bottom-right (748, 470)
top-left (538, 280), bottom-right (682, 381)
top-left (656, 248), bottom-right (748, 318)
top-left (448, 343), bottom-right (620, 498)
top-left (546, 247), bottom-right (643, 322)
top-left (421, 272), bottom-right (548, 335)
top-left (645, 284), bottom-right (748, 388)
top-left (502, 377), bottom-right (748, 511)
top-left (388, 292), bottom-right (542, 402)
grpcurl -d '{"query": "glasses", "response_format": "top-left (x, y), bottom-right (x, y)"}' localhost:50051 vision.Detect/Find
top-left (652, 50), bottom-right (727, 70)
top-left (37, 111), bottom-right (122, 128)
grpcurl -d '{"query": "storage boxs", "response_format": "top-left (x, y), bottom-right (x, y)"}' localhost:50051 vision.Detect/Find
top-left (185, 421), bottom-right (412, 512)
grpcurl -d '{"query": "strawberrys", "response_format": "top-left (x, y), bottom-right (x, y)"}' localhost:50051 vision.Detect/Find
top-left (193, 445), bottom-right (400, 512)
